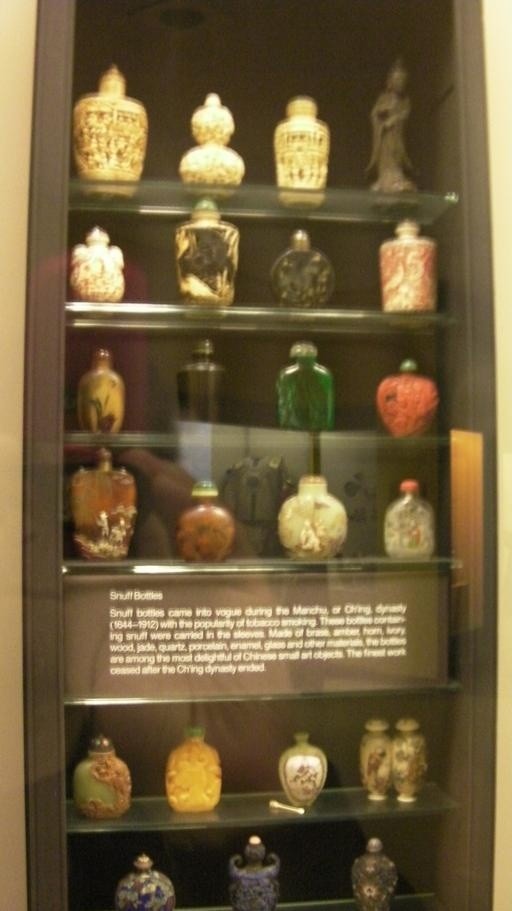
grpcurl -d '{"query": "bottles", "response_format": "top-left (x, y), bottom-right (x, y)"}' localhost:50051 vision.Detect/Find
top-left (116, 856), bottom-right (178, 911)
top-left (265, 226), bottom-right (336, 316)
top-left (374, 217), bottom-right (443, 332)
top-left (65, 339), bottom-right (442, 438)
top-left (68, 226), bottom-right (129, 322)
top-left (72, 63), bottom-right (149, 201)
top-left (173, 200), bottom-right (242, 322)
top-left (72, 712), bottom-right (428, 818)
top-left (225, 833), bottom-right (286, 911)
top-left (271, 97), bottom-right (332, 209)
top-left (350, 839), bottom-right (400, 911)
top-left (179, 92), bottom-right (247, 198)
top-left (64, 448), bottom-right (439, 565)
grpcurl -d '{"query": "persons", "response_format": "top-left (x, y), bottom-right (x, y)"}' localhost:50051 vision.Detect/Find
top-left (364, 64), bottom-right (419, 193)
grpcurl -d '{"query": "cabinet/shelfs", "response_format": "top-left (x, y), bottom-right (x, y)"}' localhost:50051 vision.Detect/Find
top-left (24, 0), bottom-right (497, 911)
